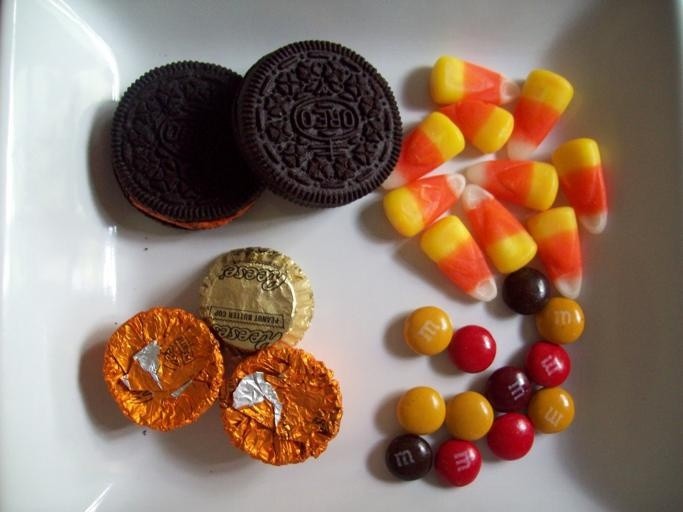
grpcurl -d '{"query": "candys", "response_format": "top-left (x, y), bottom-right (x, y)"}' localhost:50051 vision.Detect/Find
top-left (381, 55), bottom-right (609, 487)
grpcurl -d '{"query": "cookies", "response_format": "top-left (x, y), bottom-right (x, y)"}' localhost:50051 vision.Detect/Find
top-left (110, 61), bottom-right (264, 229)
top-left (231, 40), bottom-right (402, 210)
